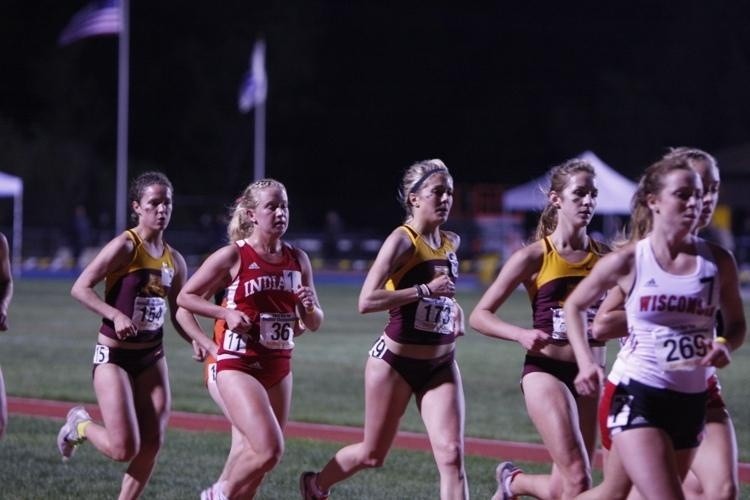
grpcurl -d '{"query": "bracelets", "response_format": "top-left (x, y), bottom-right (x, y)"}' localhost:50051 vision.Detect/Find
top-left (413, 283), bottom-right (423, 301)
top-left (422, 282), bottom-right (432, 297)
top-left (304, 305), bottom-right (316, 314)
top-left (713, 335), bottom-right (731, 349)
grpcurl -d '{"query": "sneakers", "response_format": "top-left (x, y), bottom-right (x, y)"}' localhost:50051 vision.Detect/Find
top-left (299, 472), bottom-right (331, 499)
top-left (57, 406), bottom-right (93, 459)
top-left (490, 462), bottom-right (524, 499)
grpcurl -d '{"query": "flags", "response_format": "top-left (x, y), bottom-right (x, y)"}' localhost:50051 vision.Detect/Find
top-left (57, 0), bottom-right (127, 46)
top-left (236, 36), bottom-right (268, 115)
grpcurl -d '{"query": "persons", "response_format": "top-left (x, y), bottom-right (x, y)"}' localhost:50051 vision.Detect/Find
top-left (56, 170), bottom-right (208, 499)
top-left (1, 231), bottom-right (15, 440)
top-left (175, 178), bottom-right (324, 500)
top-left (174, 193), bottom-right (256, 500)
top-left (60, 198), bottom-right (350, 274)
top-left (557, 159), bottom-right (746, 498)
top-left (468, 158), bottom-right (615, 500)
top-left (571, 178), bottom-right (654, 499)
top-left (664, 144), bottom-right (740, 499)
top-left (297, 158), bottom-right (471, 499)
